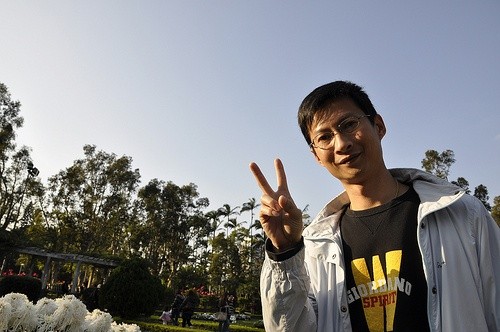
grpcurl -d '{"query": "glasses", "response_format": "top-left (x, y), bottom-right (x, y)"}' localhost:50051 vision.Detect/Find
top-left (308, 114), bottom-right (371, 148)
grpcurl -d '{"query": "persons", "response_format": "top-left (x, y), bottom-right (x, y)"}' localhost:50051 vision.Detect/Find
top-left (216, 293), bottom-right (237, 332)
top-left (248, 80), bottom-right (500, 332)
top-left (158, 289), bottom-right (197, 328)
